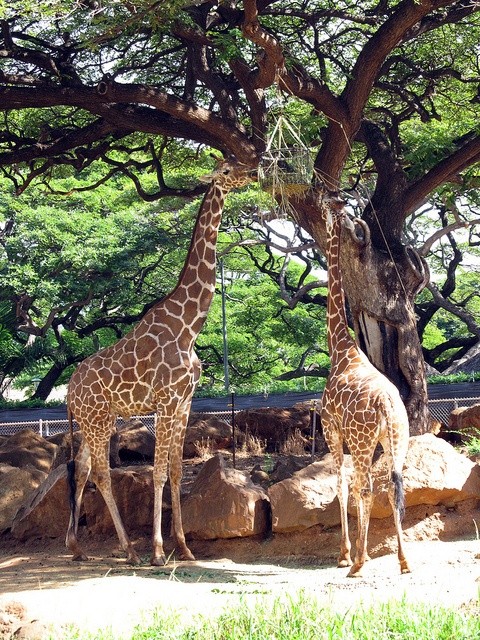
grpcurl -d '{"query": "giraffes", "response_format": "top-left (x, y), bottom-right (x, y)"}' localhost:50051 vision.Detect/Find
top-left (65, 151), bottom-right (258, 566)
top-left (316, 184), bottom-right (411, 578)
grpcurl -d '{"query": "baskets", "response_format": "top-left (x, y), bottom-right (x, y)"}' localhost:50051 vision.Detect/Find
top-left (258, 148), bottom-right (311, 195)
